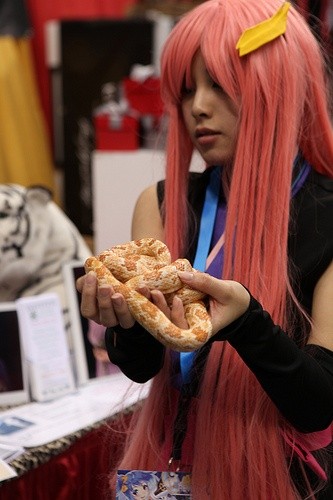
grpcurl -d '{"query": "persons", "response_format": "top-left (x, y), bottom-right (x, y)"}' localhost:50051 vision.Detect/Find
top-left (74, 0), bottom-right (333, 500)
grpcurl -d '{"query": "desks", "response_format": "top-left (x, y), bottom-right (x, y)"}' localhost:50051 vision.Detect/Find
top-left (0, 362), bottom-right (154, 500)
